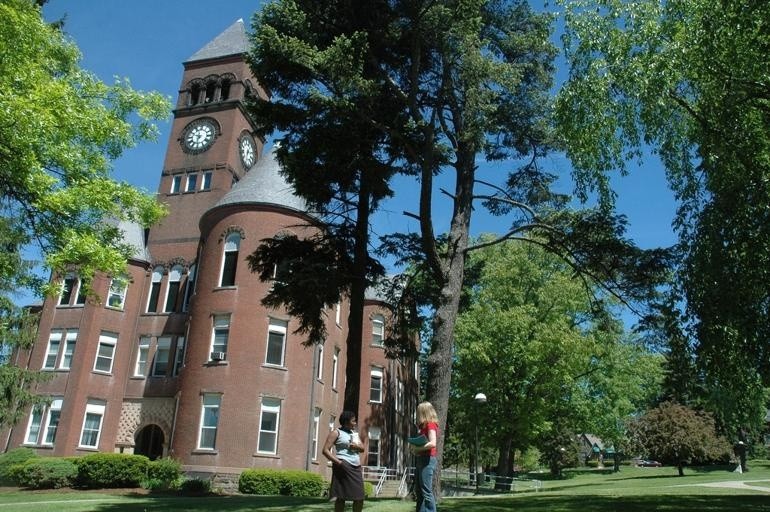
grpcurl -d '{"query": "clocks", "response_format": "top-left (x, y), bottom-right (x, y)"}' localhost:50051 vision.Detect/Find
top-left (177, 117), bottom-right (223, 155)
top-left (238, 129), bottom-right (259, 172)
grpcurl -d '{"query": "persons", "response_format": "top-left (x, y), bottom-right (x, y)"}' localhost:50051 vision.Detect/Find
top-left (412, 400), bottom-right (441, 512)
top-left (322, 411), bottom-right (367, 512)
